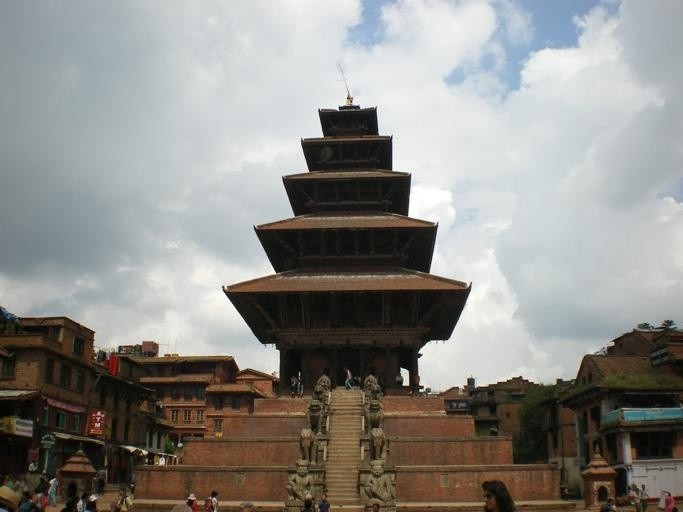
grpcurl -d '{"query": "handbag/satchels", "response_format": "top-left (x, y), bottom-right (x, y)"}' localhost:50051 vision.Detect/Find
top-left (658, 495), bottom-right (664, 510)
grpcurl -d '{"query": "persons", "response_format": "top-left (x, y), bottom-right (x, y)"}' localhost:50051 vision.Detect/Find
top-left (480, 479), bottom-right (516, 512)
top-left (289, 364), bottom-right (404, 399)
top-left (372, 503), bottom-right (380, 511)
top-left (0, 459), bottom-right (219, 512)
top-left (284, 459), bottom-right (315, 501)
top-left (363, 460), bottom-right (396, 502)
top-left (316, 492), bottom-right (330, 512)
top-left (599, 483), bottom-right (679, 511)
top-left (300, 494), bottom-right (314, 512)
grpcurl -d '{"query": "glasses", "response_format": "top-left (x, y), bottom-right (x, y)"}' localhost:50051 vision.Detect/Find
top-left (482, 493), bottom-right (494, 499)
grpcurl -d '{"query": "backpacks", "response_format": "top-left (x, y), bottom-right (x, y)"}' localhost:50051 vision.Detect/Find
top-left (204, 497), bottom-right (214, 512)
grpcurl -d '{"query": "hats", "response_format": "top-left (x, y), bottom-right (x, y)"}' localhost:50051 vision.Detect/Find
top-left (88, 495), bottom-right (96, 502)
top-left (188, 494), bottom-right (195, 500)
